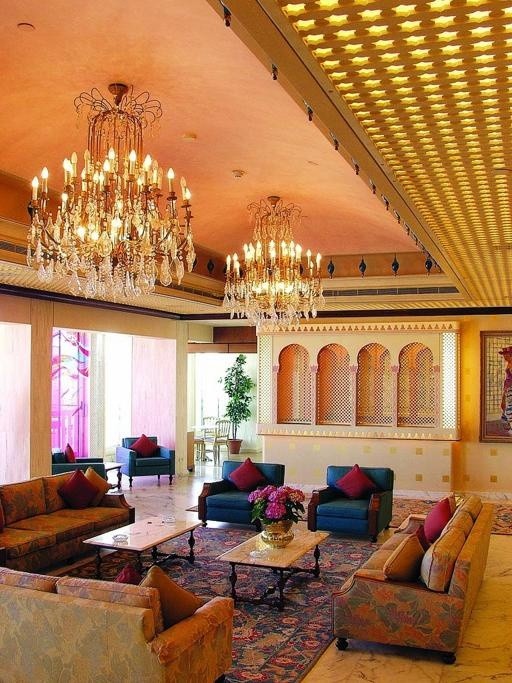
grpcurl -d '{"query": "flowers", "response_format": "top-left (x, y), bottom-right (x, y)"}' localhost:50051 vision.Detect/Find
top-left (249, 486), bottom-right (309, 529)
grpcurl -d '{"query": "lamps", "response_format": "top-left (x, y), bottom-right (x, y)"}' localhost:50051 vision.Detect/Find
top-left (223, 195), bottom-right (329, 334)
top-left (24, 86), bottom-right (195, 300)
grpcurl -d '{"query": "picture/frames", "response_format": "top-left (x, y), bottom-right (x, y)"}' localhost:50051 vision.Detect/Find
top-left (480, 331), bottom-right (511, 443)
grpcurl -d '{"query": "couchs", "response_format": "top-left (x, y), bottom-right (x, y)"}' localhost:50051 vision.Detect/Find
top-left (1, 567), bottom-right (232, 682)
top-left (115, 437), bottom-right (177, 486)
top-left (201, 461), bottom-right (286, 528)
top-left (1, 472), bottom-right (135, 575)
top-left (52, 451), bottom-right (107, 482)
top-left (332, 503), bottom-right (494, 664)
top-left (308, 466), bottom-right (393, 544)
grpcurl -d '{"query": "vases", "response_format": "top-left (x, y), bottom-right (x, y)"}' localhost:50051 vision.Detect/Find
top-left (259, 521), bottom-right (297, 549)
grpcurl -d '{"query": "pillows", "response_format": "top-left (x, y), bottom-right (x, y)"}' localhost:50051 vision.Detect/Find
top-left (129, 435), bottom-right (159, 457)
top-left (83, 468), bottom-right (111, 507)
top-left (66, 444), bottom-right (77, 463)
top-left (228, 459), bottom-right (262, 492)
top-left (108, 563), bottom-right (136, 585)
top-left (335, 464), bottom-right (378, 500)
top-left (137, 567), bottom-right (204, 628)
top-left (421, 498), bottom-right (451, 546)
top-left (382, 534), bottom-right (422, 584)
top-left (57, 470), bottom-right (95, 510)
top-left (443, 492), bottom-right (458, 517)
top-left (395, 512), bottom-right (424, 535)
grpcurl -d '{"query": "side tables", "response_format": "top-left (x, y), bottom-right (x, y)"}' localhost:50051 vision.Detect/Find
top-left (105, 461), bottom-right (126, 491)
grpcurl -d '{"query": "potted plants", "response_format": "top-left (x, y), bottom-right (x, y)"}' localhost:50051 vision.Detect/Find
top-left (218, 354), bottom-right (256, 452)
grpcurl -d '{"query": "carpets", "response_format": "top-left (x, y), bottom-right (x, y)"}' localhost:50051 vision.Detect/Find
top-left (188, 483), bottom-right (512, 536)
top-left (52, 526), bottom-right (381, 683)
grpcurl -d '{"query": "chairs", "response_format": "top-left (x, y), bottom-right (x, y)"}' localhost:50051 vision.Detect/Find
top-left (193, 415), bottom-right (230, 465)
